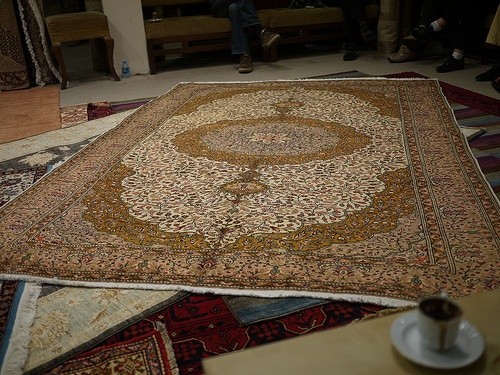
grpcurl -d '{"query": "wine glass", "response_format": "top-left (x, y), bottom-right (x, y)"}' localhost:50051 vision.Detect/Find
top-left (153, 12), bottom-right (157, 21)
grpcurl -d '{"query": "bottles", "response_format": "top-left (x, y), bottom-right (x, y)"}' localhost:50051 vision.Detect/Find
top-left (122, 61), bottom-right (129, 79)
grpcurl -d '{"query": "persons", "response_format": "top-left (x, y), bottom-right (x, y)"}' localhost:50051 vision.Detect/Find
top-left (209, 0), bottom-right (280, 72)
top-left (388, 0), bottom-right (500, 92)
top-left (339, 0), bottom-right (368, 61)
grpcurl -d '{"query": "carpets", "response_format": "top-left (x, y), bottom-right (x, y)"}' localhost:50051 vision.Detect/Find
top-left (0, 68), bottom-right (500, 375)
top-left (0, 0), bottom-right (69, 90)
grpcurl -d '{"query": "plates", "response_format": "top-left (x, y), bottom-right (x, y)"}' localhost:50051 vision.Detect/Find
top-left (391, 312), bottom-right (485, 369)
top-left (149, 19), bottom-right (162, 21)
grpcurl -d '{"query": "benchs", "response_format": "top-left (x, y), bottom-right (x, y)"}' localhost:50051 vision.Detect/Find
top-left (141, 0), bottom-right (382, 75)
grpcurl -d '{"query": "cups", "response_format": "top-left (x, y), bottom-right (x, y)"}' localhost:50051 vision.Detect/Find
top-left (418, 293), bottom-right (463, 351)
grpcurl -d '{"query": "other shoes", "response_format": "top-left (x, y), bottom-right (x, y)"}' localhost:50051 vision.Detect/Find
top-left (336, 44), bottom-right (345, 52)
top-left (342, 50), bottom-right (357, 60)
top-left (474, 62), bottom-right (500, 92)
top-left (436, 55), bottom-right (464, 72)
top-left (386, 52), bottom-right (416, 61)
top-left (238, 51), bottom-right (254, 72)
top-left (260, 28), bottom-right (280, 47)
top-left (406, 22), bottom-right (437, 39)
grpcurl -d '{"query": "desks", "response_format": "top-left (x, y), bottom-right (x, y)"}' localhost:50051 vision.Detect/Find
top-left (202, 287), bottom-right (500, 375)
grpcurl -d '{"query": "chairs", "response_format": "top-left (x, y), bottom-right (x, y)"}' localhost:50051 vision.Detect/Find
top-left (37, 0), bottom-right (120, 90)
